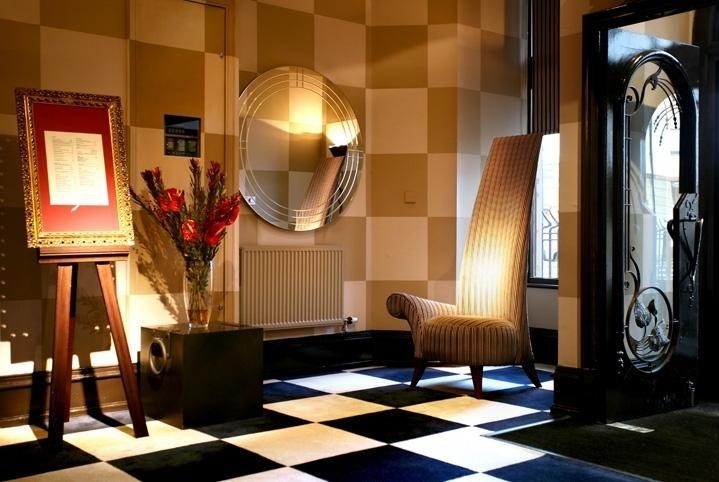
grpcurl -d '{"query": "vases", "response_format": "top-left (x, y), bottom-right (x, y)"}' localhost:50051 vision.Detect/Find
top-left (183, 261), bottom-right (213, 328)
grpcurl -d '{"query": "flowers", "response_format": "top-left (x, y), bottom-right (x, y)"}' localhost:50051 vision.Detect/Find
top-left (130, 157), bottom-right (239, 321)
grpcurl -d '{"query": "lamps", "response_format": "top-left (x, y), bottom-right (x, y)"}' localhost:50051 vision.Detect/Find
top-left (328, 145), bottom-right (348, 214)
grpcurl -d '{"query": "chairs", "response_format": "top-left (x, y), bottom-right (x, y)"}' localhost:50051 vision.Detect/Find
top-left (385, 130), bottom-right (544, 398)
top-left (294, 156), bottom-right (344, 231)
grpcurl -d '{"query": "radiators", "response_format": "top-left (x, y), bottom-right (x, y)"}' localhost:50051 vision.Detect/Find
top-left (238, 246), bottom-right (358, 333)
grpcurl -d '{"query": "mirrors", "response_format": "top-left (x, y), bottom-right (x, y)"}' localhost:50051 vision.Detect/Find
top-left (239, 65), bottom-right (363, 232)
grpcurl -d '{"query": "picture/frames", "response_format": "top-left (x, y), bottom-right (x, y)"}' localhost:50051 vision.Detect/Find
top-left (15, 87), bottom-right (135, 249)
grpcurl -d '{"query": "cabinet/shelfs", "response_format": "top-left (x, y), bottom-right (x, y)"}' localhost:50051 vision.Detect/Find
top-left (139, 321), bottom-right (263, 430)
